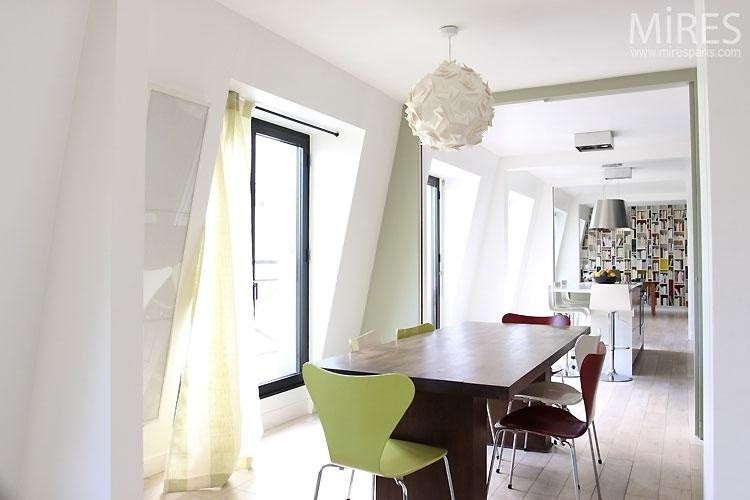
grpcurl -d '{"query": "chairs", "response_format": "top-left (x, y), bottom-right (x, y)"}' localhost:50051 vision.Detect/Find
top-left (396, 322), bottom-right (433, 339)
top-left (348, 329), bottom-right (384, 352)
top-left (301, 360), bottom-right (457, 499)
top-left (486, 342), bottom-right (607, 500)
top-left (494, 327), bottom-right (604, 473)
top-left (501, 312), bottom-right (571, 329)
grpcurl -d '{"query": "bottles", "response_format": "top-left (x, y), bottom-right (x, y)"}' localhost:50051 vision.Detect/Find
top-left (608, 266), bottom-right (622, 283)
top-left (624, 269), bottom-right (632, 284)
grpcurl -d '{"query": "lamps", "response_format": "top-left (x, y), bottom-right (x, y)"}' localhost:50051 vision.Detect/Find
top-left (404, 26), bottom-right (495, 151)
top-left (589, 199), bottom-right (631, 232)
top-left (603, 166), bottom-right (632, 180)
top-left (574, 131), bottom-right (614, 152)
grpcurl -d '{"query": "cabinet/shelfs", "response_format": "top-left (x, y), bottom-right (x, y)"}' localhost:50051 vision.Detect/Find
top-left (581, 204), bottom-right (687, 307)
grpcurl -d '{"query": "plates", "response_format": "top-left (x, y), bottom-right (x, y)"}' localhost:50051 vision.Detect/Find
top-left (594, 276), bottom-right (616, 284)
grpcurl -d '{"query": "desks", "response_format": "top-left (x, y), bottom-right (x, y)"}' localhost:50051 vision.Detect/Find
top-left (643, 281), bottom-right (659, 316)
top-left (313, 319), bottom-right (591, 500)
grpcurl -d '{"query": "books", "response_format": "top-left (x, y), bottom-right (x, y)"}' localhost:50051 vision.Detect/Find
top-left (580, 205), bottom-right (688, 307)
